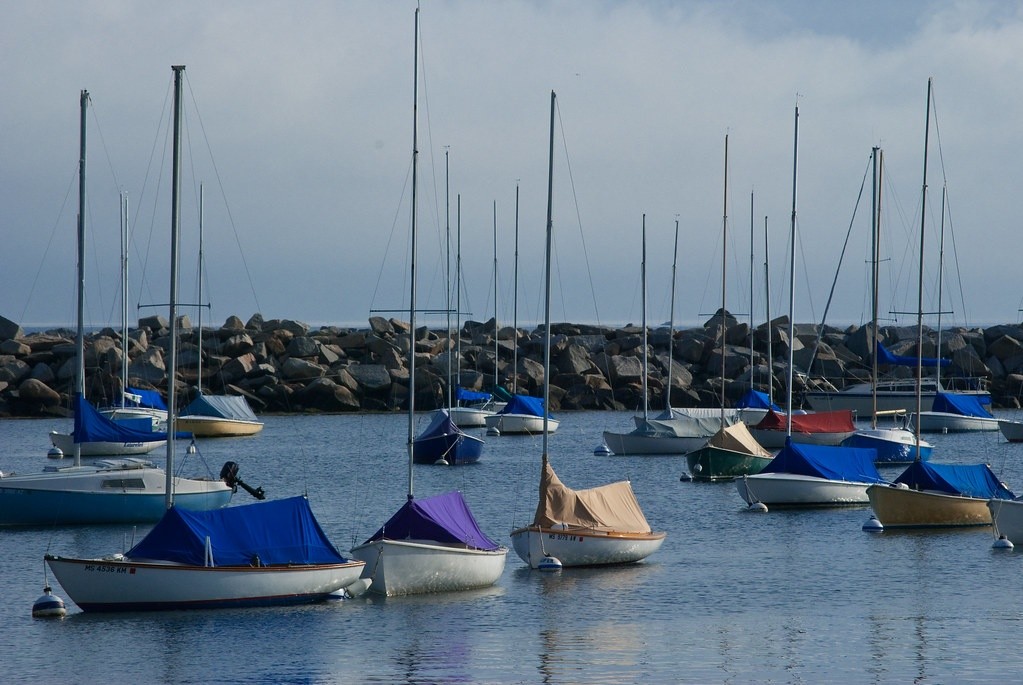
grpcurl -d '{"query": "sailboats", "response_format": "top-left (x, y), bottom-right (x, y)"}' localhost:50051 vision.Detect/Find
top-left (94, 187), bottom-right (177, 435)
top-left (600, 76), bottom-right (1023, 477)
top-left (864, 77), bottom-right (1018, 530)
top-left (49, 87), bottom-right (198, 457)
top-left (428, 192), bottom-right (510, 430)
top-left (0, 85), bottom-right (270, 529)
top-left (407, 145), bottom-right (486, 467)
top-left (44, 63), bottom-right (368, 613)
top-left (508, 89), bottom-right (668, 571)
top-left (731, 92), bottom-right (894, 512)
top-left (172, 178), bottom-right (265, 434)
top-left (483, 177), bottom-right (561, 433)
top-left (349, 0), bottom-right (513, 597)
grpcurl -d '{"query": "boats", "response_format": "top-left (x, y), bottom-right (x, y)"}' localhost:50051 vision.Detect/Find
top-left (987, 496), bottom-right (1023, 547)
